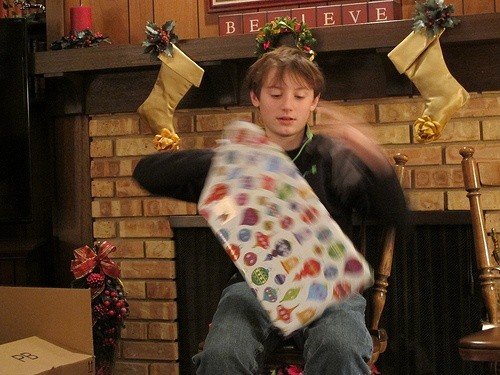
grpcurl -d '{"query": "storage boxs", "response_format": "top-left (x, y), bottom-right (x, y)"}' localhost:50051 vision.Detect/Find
top-left (197, 120), bottom-right (375, 338)
top-left (0, 287), bottom-right (96, 375)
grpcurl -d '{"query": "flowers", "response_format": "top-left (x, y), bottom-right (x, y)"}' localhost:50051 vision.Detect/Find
top-left (411, 0), bottom-right (463, 35)
top-left (254, 16), bottom-right (317, 61)
top-left (49, 28), bottom-right (111, 51)
top-left (141, 18), bottom-right (178, 60)
top-left (70, 241), bottom-right (129, 375)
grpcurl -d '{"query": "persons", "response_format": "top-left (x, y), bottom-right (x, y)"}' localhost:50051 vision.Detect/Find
top-left (132, 47), bottom-right (410, 375)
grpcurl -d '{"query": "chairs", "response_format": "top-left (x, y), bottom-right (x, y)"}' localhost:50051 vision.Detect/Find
top-left (266, 153), bottom-right (409, 375)
top-left (456, 146), bottom-right (500, 362)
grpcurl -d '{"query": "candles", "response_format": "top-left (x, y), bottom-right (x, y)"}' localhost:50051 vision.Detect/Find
top-left (70, 6), bottom-right (92, 33)
